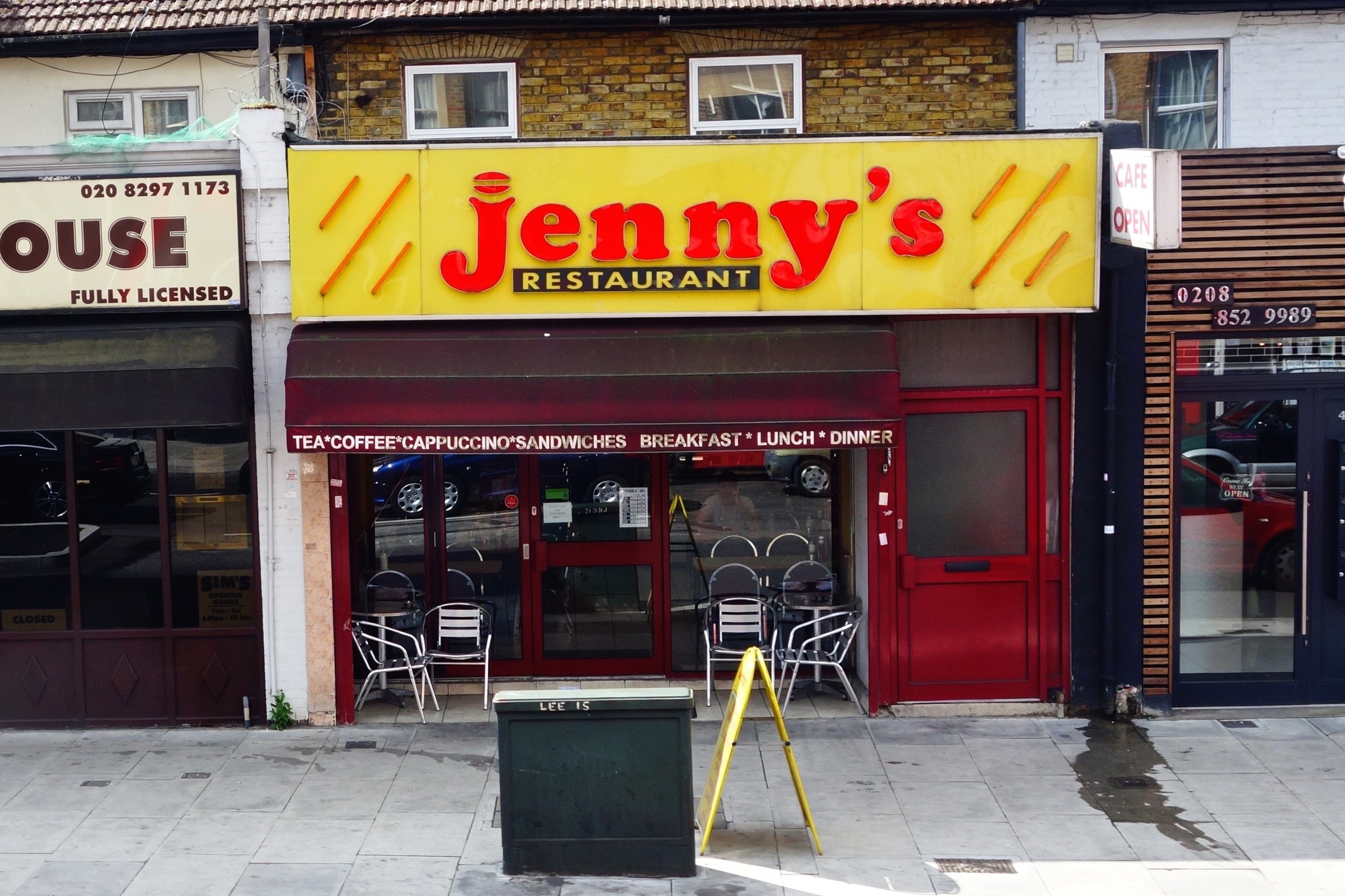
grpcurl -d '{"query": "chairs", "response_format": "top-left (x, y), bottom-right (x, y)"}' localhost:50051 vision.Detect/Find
top-left (703, 597), bottom-right (778, 707)
top-left (766, 533), bottom-right (819, 558)
top-left (776, 610), bottom-right (864, 719)
top-left (775, 560), bottom-right (836, 615)
top-left (711, 535), bottom-right (758, 557)
top-left (350, 542), bottom-right (495, 724)
top-left (708, 563), bottom-right (760, 598)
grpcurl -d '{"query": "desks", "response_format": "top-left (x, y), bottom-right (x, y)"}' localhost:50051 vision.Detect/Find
top-left (352, 601), bottom-right (422, 706)
top-left (690, 556), bottom-right (828, 590)
top-left (775, 592), bottom-right (860, 701)
top-left (377, 560), bottom-right (504, 601)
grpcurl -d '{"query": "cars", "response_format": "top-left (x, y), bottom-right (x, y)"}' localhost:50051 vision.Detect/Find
top-left (0, 431), bottom-right (153, 524)
top-left (1177, 397), bottom-right (1299, 595)
top-left (764, 449), bottom-right (838, 499)
top-left (372, 452), bottom-right (651, 520)
